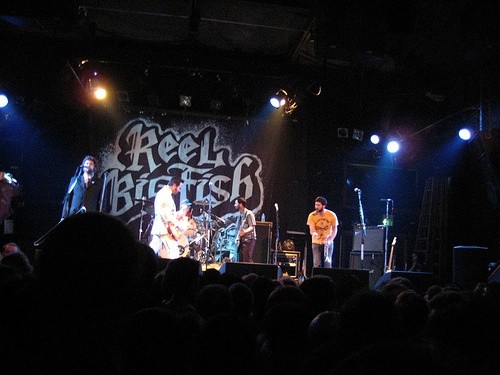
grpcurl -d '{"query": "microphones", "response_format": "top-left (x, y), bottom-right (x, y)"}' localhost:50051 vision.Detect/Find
top-left (274, 203), bottom-right (279, 210)
top-left (380, 198), bottom-right (392, 202)
top-left (354, 188), bottom-right (361, 193)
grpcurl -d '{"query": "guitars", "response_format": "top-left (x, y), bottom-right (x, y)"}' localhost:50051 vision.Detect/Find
top-left (167, 200), bottom-right (197, 241)
top-left (235, 217), bottom-right (247, 244)
top-left (386, 237), bottom-right (397, 273)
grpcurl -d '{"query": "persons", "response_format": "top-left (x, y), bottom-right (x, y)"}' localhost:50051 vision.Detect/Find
top-left (175, 198), bottom-right (205, 247)
top-left (0, 216), bottom-right (500, 375)
top-left (307, 197), bottom-right (339, 270)
top-left (61, 155), bottom-right (104, 222)
top-left (149, 176), bottom-right (189, 260)
top-left (234, 196), bottom-right (257, 263)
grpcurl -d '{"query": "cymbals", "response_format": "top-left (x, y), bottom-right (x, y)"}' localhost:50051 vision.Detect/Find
top-left (193, 200), bottom-right (216, 205)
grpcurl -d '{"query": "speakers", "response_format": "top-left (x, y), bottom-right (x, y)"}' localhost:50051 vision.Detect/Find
top-left (374, 271), bottom-right (434, 294)
top-left (349, 252), bottom-right (386, 289)
top-left (312, 267), bottom-right (370, 290)
top-left (218, 262), bottom-right (278, 280)
top-left (251, 221), bottom-right (272, 264)
top-left (453, 246), bottom-right (488, 285)
top-left (351, 224), bottom-right (386, 253)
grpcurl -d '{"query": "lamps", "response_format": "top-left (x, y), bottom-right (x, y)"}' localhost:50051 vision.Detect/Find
top-left (270, 89), bottom-right (288, 108)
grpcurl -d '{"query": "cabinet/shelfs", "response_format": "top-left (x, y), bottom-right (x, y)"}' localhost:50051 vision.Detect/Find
top-left (244, 221), bottom-right (273, 265)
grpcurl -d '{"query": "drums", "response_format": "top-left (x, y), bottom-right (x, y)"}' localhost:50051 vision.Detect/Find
top-left (178, 234), bottom-right (189, 258)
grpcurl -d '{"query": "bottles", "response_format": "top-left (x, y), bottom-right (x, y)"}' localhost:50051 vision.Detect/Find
top-left (261, 213), bottom-right (265, 223)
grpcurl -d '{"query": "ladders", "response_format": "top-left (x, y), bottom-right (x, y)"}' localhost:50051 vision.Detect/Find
top-left (414, 176), bottom-right (435, 261)
